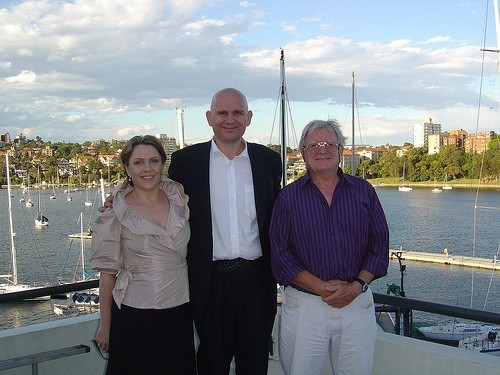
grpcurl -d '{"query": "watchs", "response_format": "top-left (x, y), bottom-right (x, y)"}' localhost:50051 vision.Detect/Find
top-left (355, 277), bottom-right (369, 293)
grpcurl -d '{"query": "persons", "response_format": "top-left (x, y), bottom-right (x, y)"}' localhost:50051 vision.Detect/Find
top-left (91, 134), bottom-right (196, 375)
top-left (269, 119), bottom-right (389, 375)
top-left (97, 87), bottom-right (282, 375)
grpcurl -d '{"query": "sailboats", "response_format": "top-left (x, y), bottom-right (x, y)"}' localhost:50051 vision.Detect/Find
top-left (0, 153), bottom-right (54, 303)
top-left (64, 178), bottom-right (84, 202)
top-left (50, 176), bottom-right (57, 200)
top-left (84, 185), bottom-right (93, 206)
top-left (25, 174), bottom-right (34, 208)
top-left (68, 177), bottom-right (108, 239)
top-left (20, 183), bottom-right (27, 202)
top-left (105, 184), bottom-right (112, 198)
top-left (398, 161), bottom-right (413, 191)
top-left (443, 173), bottom-right (453, 190)
top-left (34, 183), bottom-right (49, 226)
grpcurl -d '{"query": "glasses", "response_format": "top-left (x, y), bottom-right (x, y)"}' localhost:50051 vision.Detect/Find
top-left (303, 141), bottom-right (341, 152)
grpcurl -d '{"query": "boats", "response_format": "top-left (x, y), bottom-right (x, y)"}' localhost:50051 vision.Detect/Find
top-left (415, 321), bottom-right (500, 354)
top-left (432, 188), bottom-right (443, 193)
top-left (10, 191), bottom-right (15, 198)
